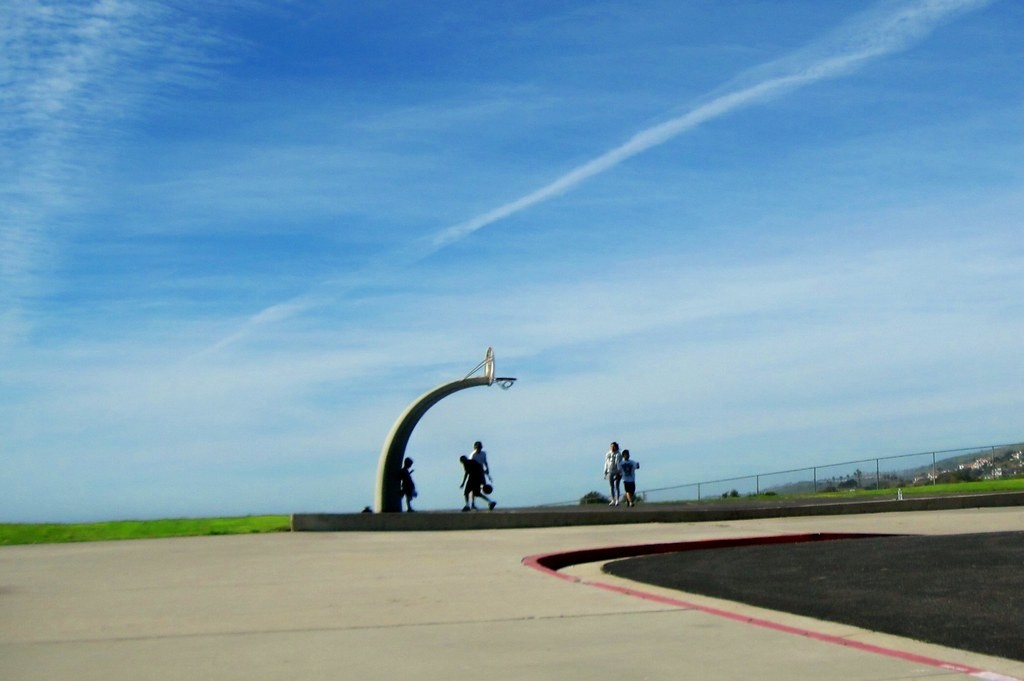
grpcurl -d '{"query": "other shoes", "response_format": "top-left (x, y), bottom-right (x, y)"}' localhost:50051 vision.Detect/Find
top-left (462, 505), bottom-right (470, 512)
top-left (407, 509), bottom-right (415, 513)
top-left (615, 500), bottom-right (619, 506)
top-left (629, 502), bottom-right (634, 507)
top-left (401, 489), bottom-right (405, 498)
top-left (609, 501), bottom-right (615, 506)
top-left (470, 508), bottom-right (477, 513)
top-left (489, 501), bottom-right (496, 510)
top-left (625, 502), bottom-right (630, 506)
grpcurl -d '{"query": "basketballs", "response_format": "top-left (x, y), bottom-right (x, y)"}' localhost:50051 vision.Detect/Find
top-left (482, 484), bottom-right (494, 495)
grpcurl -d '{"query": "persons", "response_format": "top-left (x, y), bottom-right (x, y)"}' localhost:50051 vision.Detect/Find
top-left (613, 450), bottom-right (640, 507)
top-left (459, 442), bottom-right (497, 512)
top-left (604, 442), bottom-right (623, 506)
top-left (400, 457), bottom-right (417, 512)
